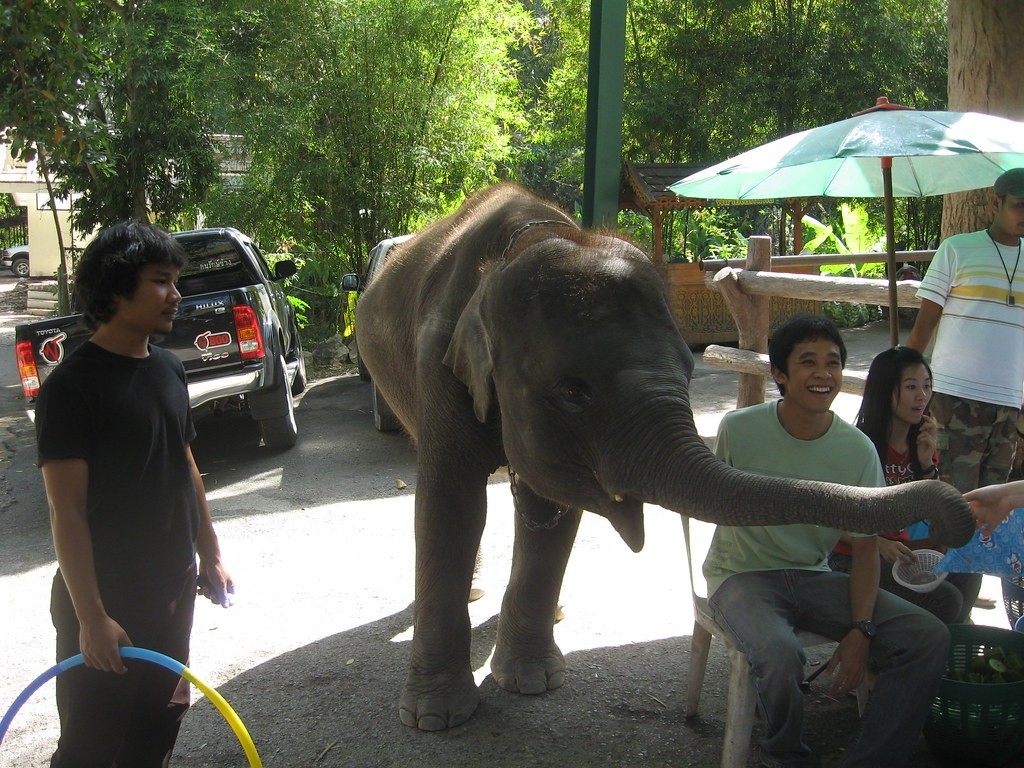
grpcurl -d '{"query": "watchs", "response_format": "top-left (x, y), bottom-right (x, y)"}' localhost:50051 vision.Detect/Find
top-left (852, 621), bottom-right (872, 638)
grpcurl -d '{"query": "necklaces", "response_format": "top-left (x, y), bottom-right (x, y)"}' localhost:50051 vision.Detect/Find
top-left (987, 229), bottom-right (1021, 307)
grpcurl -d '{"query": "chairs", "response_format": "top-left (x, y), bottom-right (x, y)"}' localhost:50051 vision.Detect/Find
top-left (680, 513), bottom-right (874, 767)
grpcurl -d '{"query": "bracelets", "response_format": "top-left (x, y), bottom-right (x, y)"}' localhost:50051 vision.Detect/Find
top-left (922, 465), bottom-right (938, 480)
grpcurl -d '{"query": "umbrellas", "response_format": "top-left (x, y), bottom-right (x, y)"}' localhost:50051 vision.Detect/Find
top-left (667, 98), bottom-right (1024, 346)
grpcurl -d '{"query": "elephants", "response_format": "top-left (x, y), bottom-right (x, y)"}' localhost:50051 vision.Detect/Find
top-left (356, 178), bottom-right (981, 734)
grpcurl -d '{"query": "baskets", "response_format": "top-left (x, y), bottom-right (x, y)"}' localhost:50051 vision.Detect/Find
top-left (891, 549), bottom-right (949, 593)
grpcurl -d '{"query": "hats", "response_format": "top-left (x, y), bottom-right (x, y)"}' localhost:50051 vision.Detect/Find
top-left (994, 168), bottom-right (1024, 198)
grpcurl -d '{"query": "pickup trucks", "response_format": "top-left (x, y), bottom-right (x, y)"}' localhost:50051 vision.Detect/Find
top-left (17, 228), bottom-right (306, 454)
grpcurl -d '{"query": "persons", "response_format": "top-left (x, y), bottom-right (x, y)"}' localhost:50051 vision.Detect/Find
top-left (963, 479), bottom-right (1024, 536)
top-left (905, 168), bottom-right (1024, 495)
top-left (35, 223), bottom-right (237, 768)
top-left (703, 312), bottom-right (950, 768)
top-left (826, 347), bottom-right (983, 626)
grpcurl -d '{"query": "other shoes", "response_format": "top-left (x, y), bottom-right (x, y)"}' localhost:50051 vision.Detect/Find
top-left (842, 676), bottom-right (873, 698)
